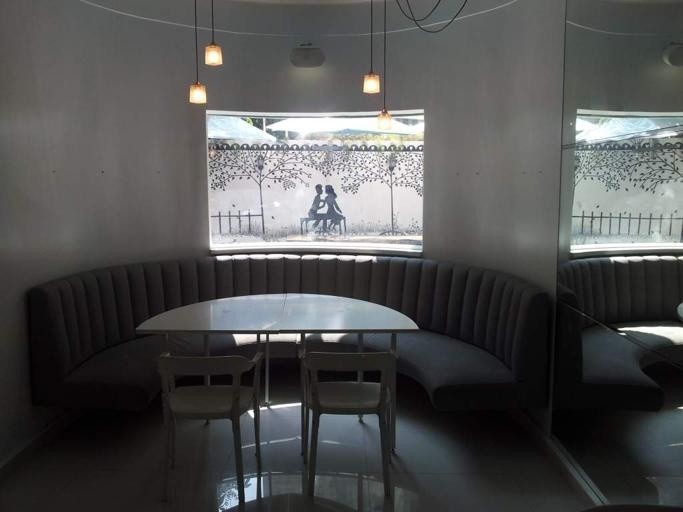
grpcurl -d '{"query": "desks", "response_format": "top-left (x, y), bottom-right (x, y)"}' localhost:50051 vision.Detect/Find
top-left (137, 293), bottom-right (420, 452)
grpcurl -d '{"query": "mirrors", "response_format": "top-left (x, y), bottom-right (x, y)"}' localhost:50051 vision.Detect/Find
top-left (550, 0), bottom-right (683, 512)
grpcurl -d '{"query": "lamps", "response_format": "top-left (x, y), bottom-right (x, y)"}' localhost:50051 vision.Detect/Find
top-left (378, 0), bottom-right (392, 130)
top-left (204, 0), bottom-right (223, 66)
top-left (363, 0), bottom-right (381, 93)
top-left (189, 0), bottom-right (206, 104)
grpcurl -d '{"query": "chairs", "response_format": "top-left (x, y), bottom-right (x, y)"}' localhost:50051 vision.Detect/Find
top-left (158, 350), bottom-right (262, 503)
top-left (299, 351), bottom-right (396, 498)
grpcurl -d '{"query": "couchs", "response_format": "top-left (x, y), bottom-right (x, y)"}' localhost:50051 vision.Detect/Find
top-left (557, 256), bottom-right (683, 412)
top-left (26, 254), bottom-right (548, 422)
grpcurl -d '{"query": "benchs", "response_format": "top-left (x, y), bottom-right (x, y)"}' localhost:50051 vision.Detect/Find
top-left (300, 216), bottom-right (346, 235)
